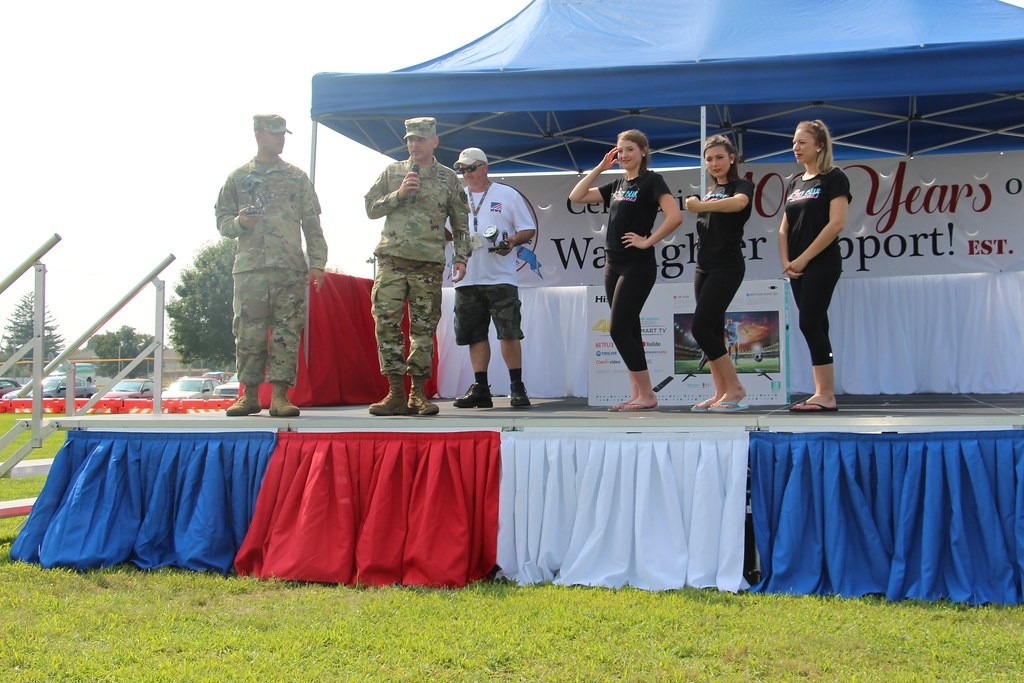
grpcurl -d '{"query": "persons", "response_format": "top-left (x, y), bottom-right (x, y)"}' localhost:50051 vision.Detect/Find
top-left (215, 114), bottom-right (327, 417)
top-left (569, 130), bottom-right (683, 411)
top-left (365, 117), bottom-right (470, 414)
top-left (778, 121), bottom-right (852, 412)
top-left (684, 134), bottom-right (756, 412)
top-left (442, 147), bottom-right (536, 409)
top-left (724, 317), bottom-right (748, 365)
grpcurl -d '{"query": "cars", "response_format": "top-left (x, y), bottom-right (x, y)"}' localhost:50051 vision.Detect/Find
top-left (201, 371), bottom-right (231, 384)
top-left (160, 376), bottom-right (224, 400)
top-left (212, 372), bottom-right (240, 400)
top-left (1, 375), bottom-right (98, 400)
top-left (100, 378), bottom-right (163, 400)
top-left (0, 377), bottom-right (22, 400)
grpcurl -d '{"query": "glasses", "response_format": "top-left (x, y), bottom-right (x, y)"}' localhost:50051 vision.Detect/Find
top-left (459, 162), bottom-right (486, 175)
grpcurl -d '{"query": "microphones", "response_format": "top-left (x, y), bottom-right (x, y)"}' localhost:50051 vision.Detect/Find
top-left (410, 163), bottom-right (419, 203)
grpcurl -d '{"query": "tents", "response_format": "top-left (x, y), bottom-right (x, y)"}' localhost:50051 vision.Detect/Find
top-left (305, 1), bottom-right (1024, 368)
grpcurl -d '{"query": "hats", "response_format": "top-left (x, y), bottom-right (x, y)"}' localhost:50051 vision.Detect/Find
top-left (253, 114), bottom-right (293, 136)
top-left (403, 117), bottom-right (437, 141)
top-left (453, 147), bottom-right (488, 169)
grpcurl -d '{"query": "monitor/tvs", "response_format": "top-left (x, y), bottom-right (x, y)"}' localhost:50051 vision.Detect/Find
top-left (673, 311), bottom-right (780, 383)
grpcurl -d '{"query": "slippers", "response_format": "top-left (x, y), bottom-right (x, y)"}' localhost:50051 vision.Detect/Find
top-left (618, 402), bottom-right (656, 412)
top-left (608, 402), bottom-right (627, 411)
top-left (691, 400), bottom-right (717, 412)
top-left (789, 401), bottom-right (838, 412)
top-left (707, 401), bottom-right (749, 413)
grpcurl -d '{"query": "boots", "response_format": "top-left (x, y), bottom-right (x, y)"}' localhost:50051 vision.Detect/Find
top-left (226, 384), bottom-right (261, 416)
top-left (369, 375), bottom-right (409, 416)
top-left (269, 381), bottom-right (299, 417)
top-left (409, 374), bottom-right (439, 415)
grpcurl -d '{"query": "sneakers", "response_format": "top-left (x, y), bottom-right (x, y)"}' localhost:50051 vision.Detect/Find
top-left (510, 379), bottom-right (530, 407)
top-left (454, 383), bottom-right (493, 408)
